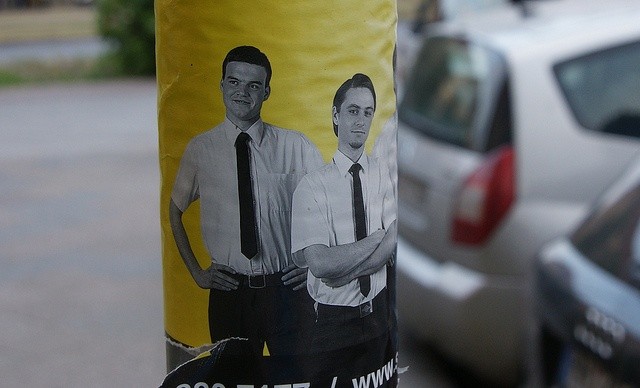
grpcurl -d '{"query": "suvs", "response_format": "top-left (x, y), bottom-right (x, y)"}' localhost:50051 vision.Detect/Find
top-left (396, 0), bottom-right (640, 380)
top-left (520, 148), bottom-right (640, 388)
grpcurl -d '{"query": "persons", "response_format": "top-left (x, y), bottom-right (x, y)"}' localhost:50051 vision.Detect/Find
top-left (169, 45), bottom-right (329, 344)
top-left (292, 73), bottom-right (398, 318)
top-left (373, 42), bottom-right (398, 192)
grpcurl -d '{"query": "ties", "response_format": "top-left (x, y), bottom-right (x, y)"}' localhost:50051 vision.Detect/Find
top-left (350, 163), bottom-right (370, 299)
top-left (234, 132), bottom-right (260, 260)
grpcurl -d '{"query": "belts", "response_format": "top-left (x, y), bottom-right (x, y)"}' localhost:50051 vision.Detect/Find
top-left (219, 270), bottom-right (289, 290)
top-left (306, 286), bottom-right (386, 318)
